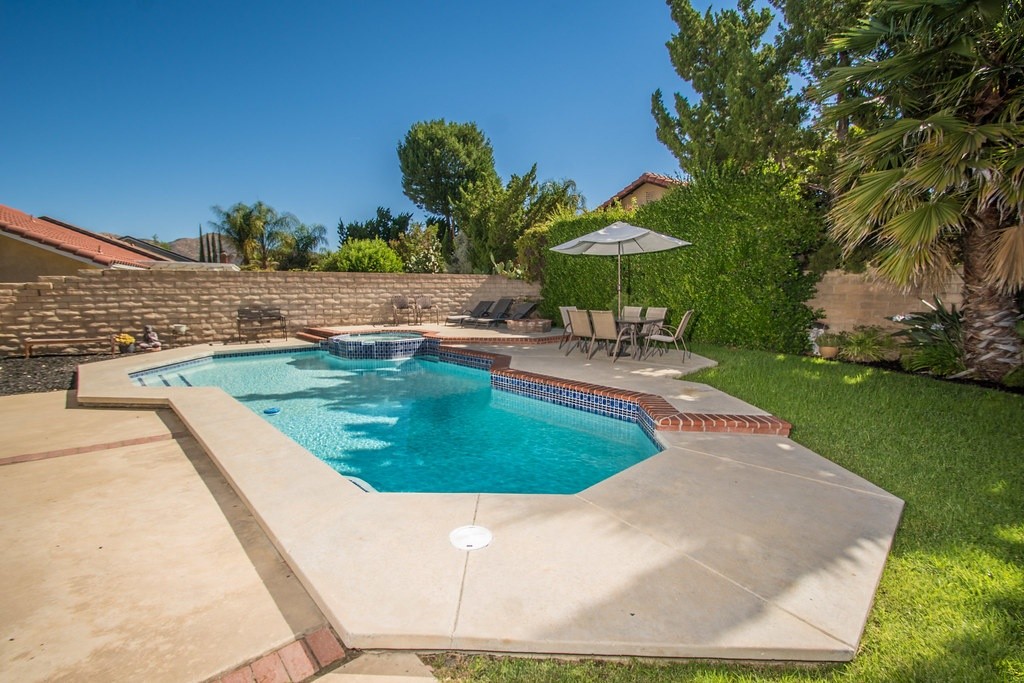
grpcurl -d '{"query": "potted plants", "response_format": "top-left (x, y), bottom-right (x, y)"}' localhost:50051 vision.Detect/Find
top-left (815, 332), bottom-right (841, 358)
top-left (119, 344), bottom-right (134, 353)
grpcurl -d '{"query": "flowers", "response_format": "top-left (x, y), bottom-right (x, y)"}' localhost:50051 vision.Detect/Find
top-left (115, 334), bottom-right (135, 345)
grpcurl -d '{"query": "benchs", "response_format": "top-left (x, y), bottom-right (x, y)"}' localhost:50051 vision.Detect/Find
top-left (24, 334), bottom-right (115, 358)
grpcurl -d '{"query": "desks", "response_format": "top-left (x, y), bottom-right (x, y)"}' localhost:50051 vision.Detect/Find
top-left (615, 316), bottom-right (665, 359)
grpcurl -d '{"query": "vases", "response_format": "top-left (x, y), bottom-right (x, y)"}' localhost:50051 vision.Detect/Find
top-left (174, 325), bottom-right (186, 335)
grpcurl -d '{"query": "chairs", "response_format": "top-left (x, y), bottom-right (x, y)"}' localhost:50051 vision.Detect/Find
top-left (391, 295), bottom-right (439, 326)
top-left (238, 308), bottom-right (287, 344)
top-left (445, 299), bottom-right (539, 329)
top-left (559, 306), bottom-right (694, 364)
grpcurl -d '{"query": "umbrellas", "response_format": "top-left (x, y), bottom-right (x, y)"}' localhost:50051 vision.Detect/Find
top-left (549, 220), bottom-right (694, 322)
